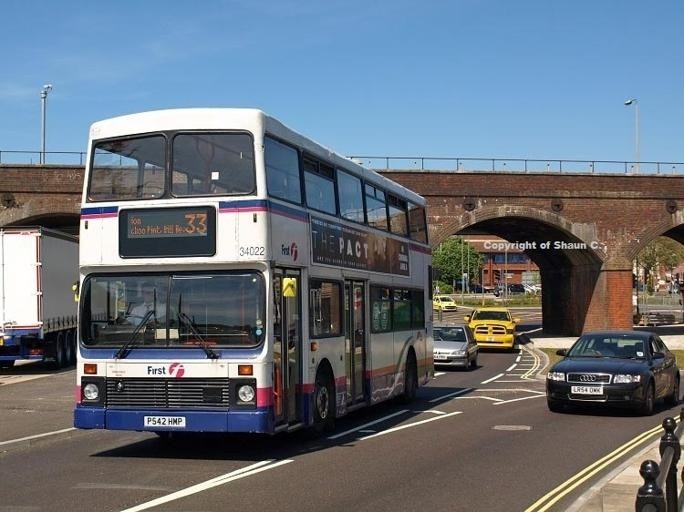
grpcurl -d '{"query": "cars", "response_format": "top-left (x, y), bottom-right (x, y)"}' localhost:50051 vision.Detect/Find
top-left (431, 323), bottom-right (479, 369)
top-left (544, 329), bottom-right (678, 414)
top-left (465, 283), bottom-right (541, 296)
top-left (432, 295), bottom-right (457, 312)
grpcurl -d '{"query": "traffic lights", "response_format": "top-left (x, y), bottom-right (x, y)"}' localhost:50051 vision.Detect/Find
top-left (644, 269), bottom-right (649, 284)
top-left (632, 273), bottom-right (638, 288)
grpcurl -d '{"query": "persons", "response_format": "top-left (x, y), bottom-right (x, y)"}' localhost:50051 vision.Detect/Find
top-left (119, 282), bottom-right (177, 327)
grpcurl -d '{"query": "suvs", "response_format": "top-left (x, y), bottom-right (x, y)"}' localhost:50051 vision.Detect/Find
top-left (463, 308), bottom-right (519, 352)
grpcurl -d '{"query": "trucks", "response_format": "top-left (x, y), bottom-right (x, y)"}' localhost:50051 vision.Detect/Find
top-left (0, 226), bottom-right (120, 374)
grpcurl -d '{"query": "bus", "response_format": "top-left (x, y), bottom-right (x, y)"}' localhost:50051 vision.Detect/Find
top-left (70, 107), bottom-right (437, 441)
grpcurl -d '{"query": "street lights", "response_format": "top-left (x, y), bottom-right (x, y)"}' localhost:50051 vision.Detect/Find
top-left (624, 97), bottom-right (640, 172)
top-left (39, 83), bottom-right (52, 164)
